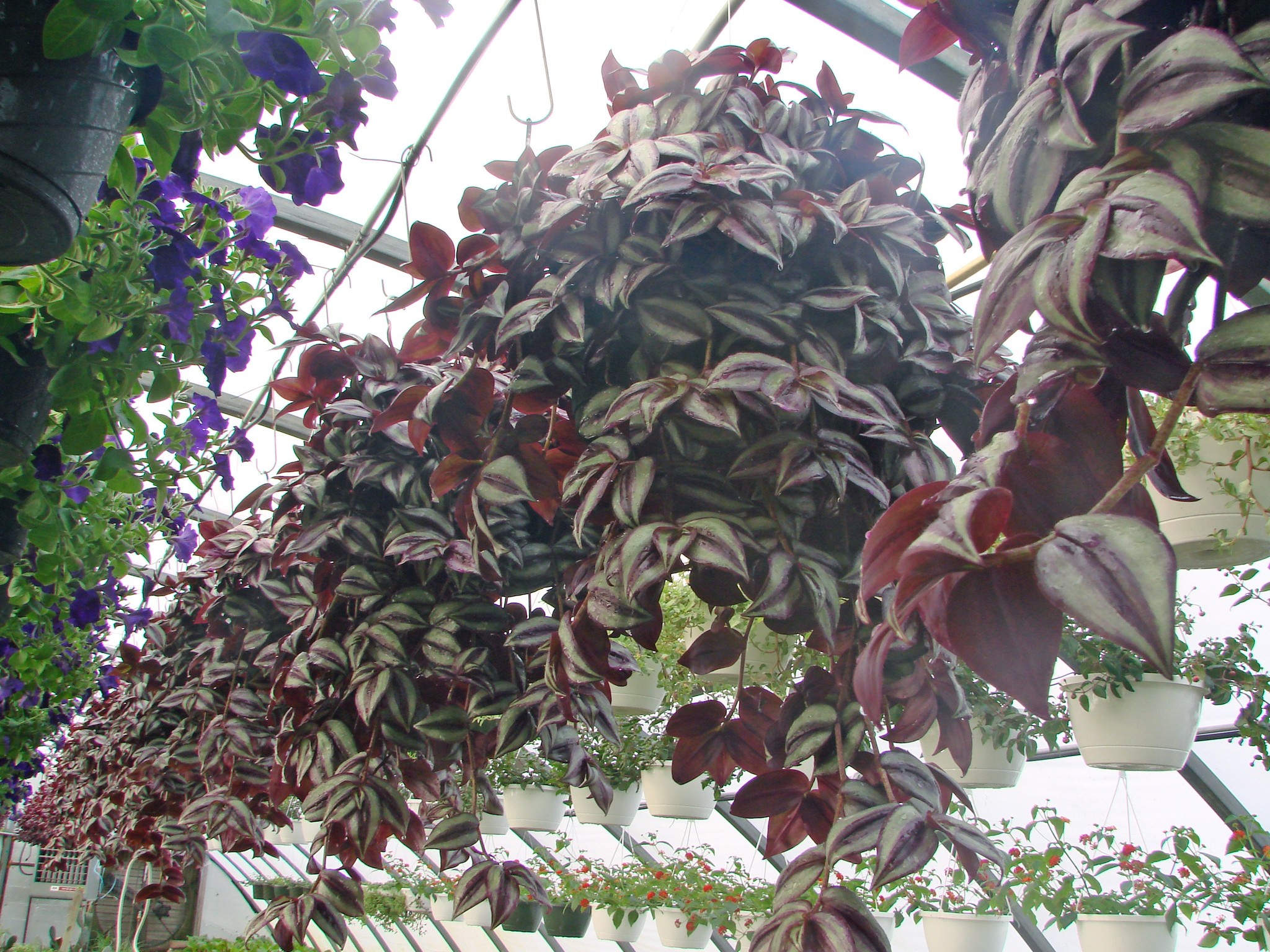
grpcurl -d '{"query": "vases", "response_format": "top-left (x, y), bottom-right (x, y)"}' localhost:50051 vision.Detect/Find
top-left (732, 911), bottom-right (784, 952)
top-left (0, 10), bottom-right (162, 267)
top-left (1070, 912), bottom-right (1176, 950)
top-left (463, 673), bottom-right (1217, 834)
top-left (244, 882), bottom-right (713, 948)
top-left (0, 334), bottom-right (61, 459)
top-left (905, 907), bottom-right (1012, 952)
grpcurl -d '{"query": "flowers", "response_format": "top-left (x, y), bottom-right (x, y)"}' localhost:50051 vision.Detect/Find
top-left (550, 829), bottom-right (667, 927)
top-left (376, 849), bottom-right (423, 890)
top-left (853, 797), bottom-right (1015, 916)
top-left (449, 624), bottom-right (1269, 808)
top-left (985, 803), bottom-right (1192, 914)
top-left (524, 853), bottom-right (592, 910)
top-left (0, 1), bottom-right (451, 828)
top-left (414, 860), bottom-right (463, 898)
top-left (680, 849), bottom-right (779, 941)
top-left (629, 832), bottom-right (717, 933)
